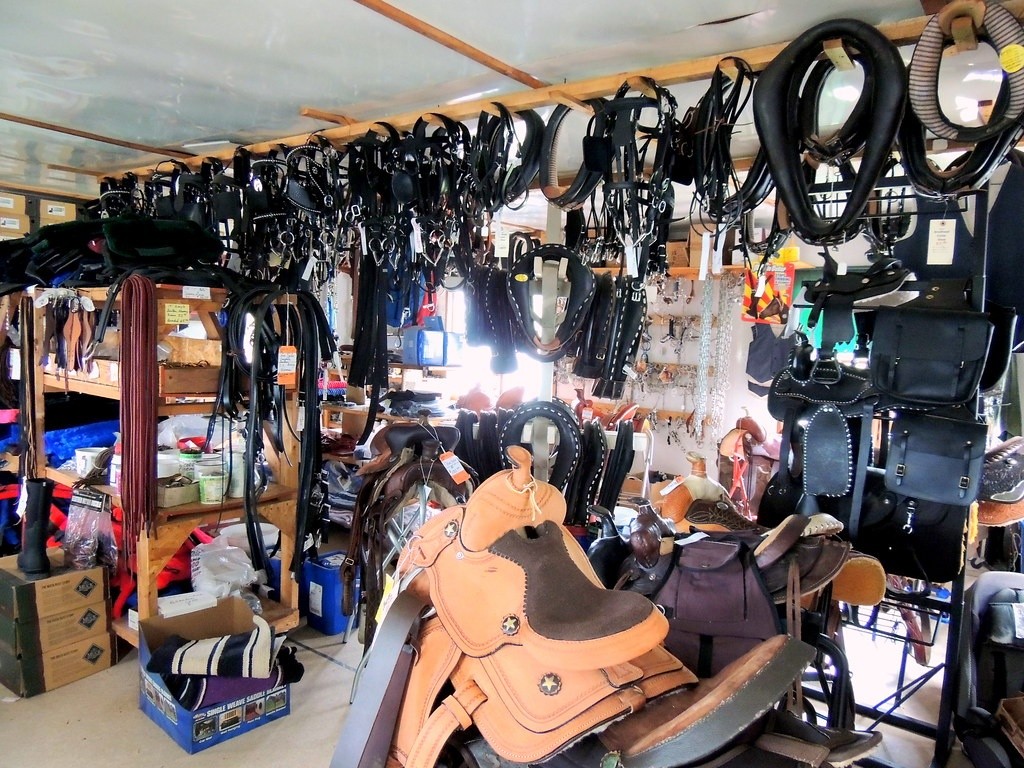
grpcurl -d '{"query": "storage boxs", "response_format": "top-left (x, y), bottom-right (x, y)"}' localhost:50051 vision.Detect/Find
top-left (0, 192), bottom-right (77, 239)
top-left (664, 241), bottom-right (691, 268)
top-left (587, 229), bottom-right (628, 268)
top-left (1, 545), bottom-right (117, 700)
top-left (689, 223), bottom-right (737, 267)
top-left (735, 228), bottom-right (801, 261)
top-left (136, 647), bottom-right (291, 755)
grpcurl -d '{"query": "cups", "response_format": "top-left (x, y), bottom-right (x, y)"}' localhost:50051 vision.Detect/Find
top-left (75, 448), bottom-right (109, 479)
top-left (179, 454), bottom-right (201, 479)
top-left (202, 453), bottom-right (222, 460)
top-left (194, 461), bottom-right (228, 505)
top-left (158, 459), bottom-right (179, 479)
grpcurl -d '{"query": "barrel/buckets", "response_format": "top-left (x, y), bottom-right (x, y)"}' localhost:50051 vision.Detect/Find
top-left (220, 523), bottom-right (279, 562)
top-left (304, 550), bottom-right (360, 635)
top-left (265, 546), bottom-right (310, 618)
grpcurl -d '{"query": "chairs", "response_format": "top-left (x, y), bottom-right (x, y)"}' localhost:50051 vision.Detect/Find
top-left (954, 571), bottom-right (1024, 768)
top-left (435, 401), bottom-right (655, 528)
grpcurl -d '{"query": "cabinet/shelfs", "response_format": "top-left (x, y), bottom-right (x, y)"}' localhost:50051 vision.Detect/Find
top-left (552, 267), bottom-right (748, 432)
top-left (0, 284), bottom-right (303, 651)
top-left (321, 353), bottom-right (455, 470)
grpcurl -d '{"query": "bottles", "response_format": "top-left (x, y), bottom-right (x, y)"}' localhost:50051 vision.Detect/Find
top-left (110, 443), bottom-right (121, 487)
